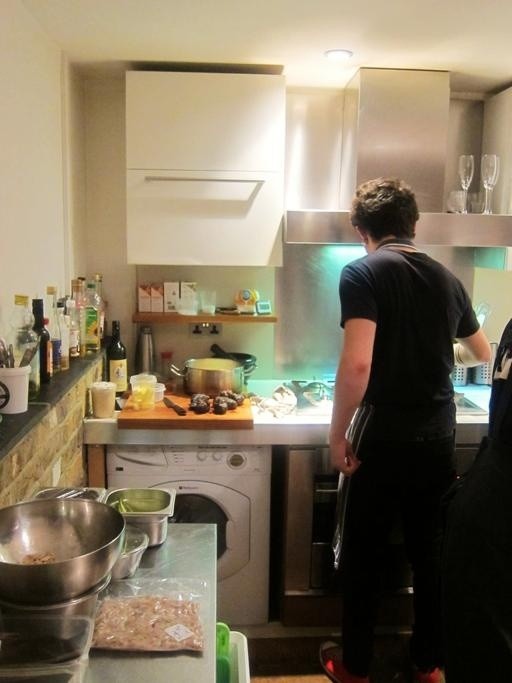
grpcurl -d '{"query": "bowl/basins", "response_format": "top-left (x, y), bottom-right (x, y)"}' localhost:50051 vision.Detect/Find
top-left (0, 570), bottom-right (113, 668)
top-left (111, 524), bottom-right (150, 580)
top-left (1, 500), bottom-right (126, 606)
top-left (211, 350), bottom-right (256, 384)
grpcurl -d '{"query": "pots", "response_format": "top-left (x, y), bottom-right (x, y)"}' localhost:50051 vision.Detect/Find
top-left (170, 358), bottom-right (257, 391)
top-left (296, 381), bottom-right (336, 411)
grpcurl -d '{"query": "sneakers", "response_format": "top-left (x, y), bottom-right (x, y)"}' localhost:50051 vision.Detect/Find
top-left (319, 640), bottom-right (369, 682)
top-left (393, 666), bottom-right (442, 682)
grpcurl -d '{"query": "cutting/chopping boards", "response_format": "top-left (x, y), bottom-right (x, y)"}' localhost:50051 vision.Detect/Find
top-left (116, 386), bottom-right (252, 429)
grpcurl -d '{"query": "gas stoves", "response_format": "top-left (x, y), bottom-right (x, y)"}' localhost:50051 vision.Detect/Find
top-left (286, 379), bottom-right (480, 412)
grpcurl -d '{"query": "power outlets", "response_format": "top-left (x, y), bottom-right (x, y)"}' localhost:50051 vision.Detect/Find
top-left (189, 323), bottom-right (222, 336)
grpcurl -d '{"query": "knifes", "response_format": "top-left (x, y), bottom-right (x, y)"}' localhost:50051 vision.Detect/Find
top-left (162, 395), bottom-right (186, 414)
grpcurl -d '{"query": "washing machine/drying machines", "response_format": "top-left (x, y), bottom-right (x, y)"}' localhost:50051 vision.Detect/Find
top-left (104, 444), bottom-right (269, 626)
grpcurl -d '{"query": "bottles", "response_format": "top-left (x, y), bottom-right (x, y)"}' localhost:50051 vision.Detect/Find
top-left (136, 326), bottom-right (156, 373)
top-left (160, 350), bottom-right (173, 378)
top-left (108, 319), bottom-right (127, 398)
top-left (8, 272), bottom-right (105, 392)
top-left (130, 374), bottom-right (156, 409)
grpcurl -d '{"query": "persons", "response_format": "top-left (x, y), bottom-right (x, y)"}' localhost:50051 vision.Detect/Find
top-left (486, 318), bottom-right (511, 679)
top-left (317, 175), bottom-right (493, 683)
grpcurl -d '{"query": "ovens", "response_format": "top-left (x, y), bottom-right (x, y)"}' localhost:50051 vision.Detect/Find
top-left (312, 478), bottom-right (415, 591)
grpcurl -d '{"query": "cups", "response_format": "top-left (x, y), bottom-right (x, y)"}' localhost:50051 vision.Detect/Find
top-left (154, 383), bottom-right (167, 402)
top-left (91, 380), bottom-right (116, 417)
top-left (200, 288), bottom-right (216, 313)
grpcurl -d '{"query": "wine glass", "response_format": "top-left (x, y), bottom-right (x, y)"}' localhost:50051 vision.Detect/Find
top-left (458, 155), bottom-right (473, 213)
top-left (480, 153), bottom-right (500, 214)
